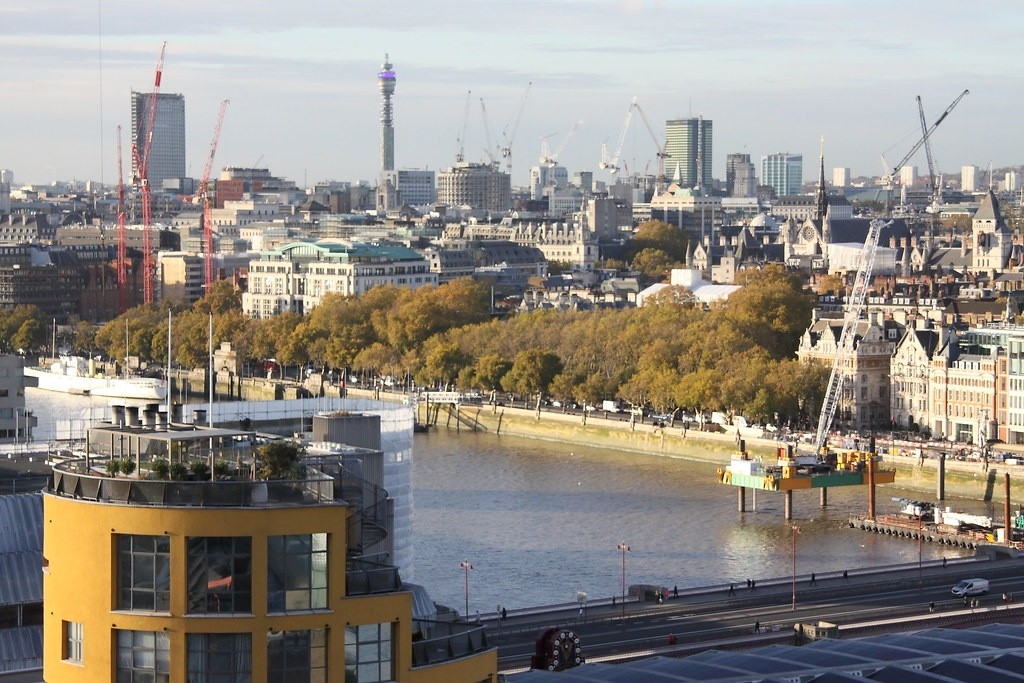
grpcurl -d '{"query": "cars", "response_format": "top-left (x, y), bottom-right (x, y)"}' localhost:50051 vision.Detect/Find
top-left (960, 524), bottom-right (984, 531)
top-left (552, 400), bottom-right (598, 411)
top-left (752, 423), bottom-right (778, 432)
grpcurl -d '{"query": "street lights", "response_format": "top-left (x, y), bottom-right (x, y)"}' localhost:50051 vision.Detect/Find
top-left (918, 510), bottom-right (926, 590)
top-left (789, 522), bottom-right (802, 608)
top-left (618, 540), bottom-right (631, 621)
top-left (460, 559), bottom-right (474, 620)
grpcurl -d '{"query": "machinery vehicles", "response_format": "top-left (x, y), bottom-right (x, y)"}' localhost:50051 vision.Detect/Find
top-left (875, 90), bottom-right (972, 214)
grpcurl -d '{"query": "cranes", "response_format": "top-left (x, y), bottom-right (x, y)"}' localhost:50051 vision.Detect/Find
top-left (537, 120), bottom-right (587, 168)
top-left (696, 115), bottom-right (706, 187)
top-left (115, 124), bottom-right (131, 314)
top-left (635, 106), bottom-right (672, 176)
top-left (195, 99), bottom-right (234, 296)
top-left (478, 97), bottom-right (508, 172)
top-left (812, 218), bottom-right (895, 459)
top-left (132, 41), bottom-right (193, 305)
top-left (456, 89), bottom-right (472, 164)
top-left (600, 95), bottom-right (640, 174)
top-left (497, 81), bottom-right (531, 173)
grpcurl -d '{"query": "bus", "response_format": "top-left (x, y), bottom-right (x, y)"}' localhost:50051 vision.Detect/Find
top-left (260, 358), bottom-right (278, 374)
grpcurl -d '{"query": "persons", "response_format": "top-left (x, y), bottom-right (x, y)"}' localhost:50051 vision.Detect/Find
top-left (754, 619), bottom-right (760, 634)
top-left (943, 557), bottom-right (947, 568)
top-left (809, 572), bottom-right (816, 587)
top-left (747, 579), bottom-right (751, 592)
top-left (1003, 591), bottom-right (1008, 605)
top-left (502, 607), bottom-right (507, 621)
top-left (475, 610), bottom-right (481, 623)
top-left (962, 596), bottom-right (969, 608)
top-left (673, 585), bottom-right (679, 599)
top-left (843, 570), bottom-right (849, 584)
top-left (654, 587), bottom-right (669, 604)
top-left (970, 597), bottom-right (976, 608)
top-left (929, 601), bottom-right (935, 614)
top-left (728, 583), bottom-right (737, 597)
top-left (611, 595), bottom-right (617, 609)
top-left (751, 580), bottom-right (757, 592)
top-left (669, 633), bottom-right (673, 644)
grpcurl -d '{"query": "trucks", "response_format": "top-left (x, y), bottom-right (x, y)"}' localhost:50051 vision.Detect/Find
top-left (603, 400), bottom-right (668, 420)
top-left (712, 412), bottom-right (748, 428)
top-left (952, 578), bottom-right (989, 597)
